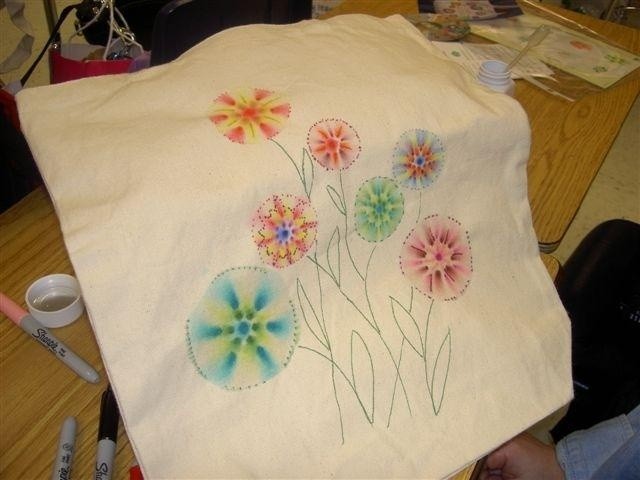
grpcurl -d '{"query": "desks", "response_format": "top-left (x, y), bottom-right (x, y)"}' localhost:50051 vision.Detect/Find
top-left (0, 183), bottom-right (566, 480)
top-left (317, 0), bottom-right (639, 252)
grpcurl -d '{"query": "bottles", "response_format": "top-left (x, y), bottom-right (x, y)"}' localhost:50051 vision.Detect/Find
top-left (476, 61), bottom-right (515, 99)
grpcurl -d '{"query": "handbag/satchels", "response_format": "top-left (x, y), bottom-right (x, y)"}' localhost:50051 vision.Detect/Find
top-left (48, 3), bottom-right (151, 82)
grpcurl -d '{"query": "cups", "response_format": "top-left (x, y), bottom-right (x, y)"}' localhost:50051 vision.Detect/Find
top-left (25, 274), bottom-right (84, 328)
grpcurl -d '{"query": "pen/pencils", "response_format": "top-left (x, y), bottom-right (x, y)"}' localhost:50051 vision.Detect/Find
top-left (52, 417), bottom-right (77, 480)
top-left (0, 293), bottom-right (101, 383)
top-left (129, 458), bottom-right (144, 480)
top-left (95, 382), bottom-right (120, 480)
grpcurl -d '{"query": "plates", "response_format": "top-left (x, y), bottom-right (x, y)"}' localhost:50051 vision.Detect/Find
top-left (407, 12), bottom-right (469, 42)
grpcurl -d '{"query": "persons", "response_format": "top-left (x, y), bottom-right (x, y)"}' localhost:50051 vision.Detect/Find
top-left (477, 404), bottom-right (640, 480)
